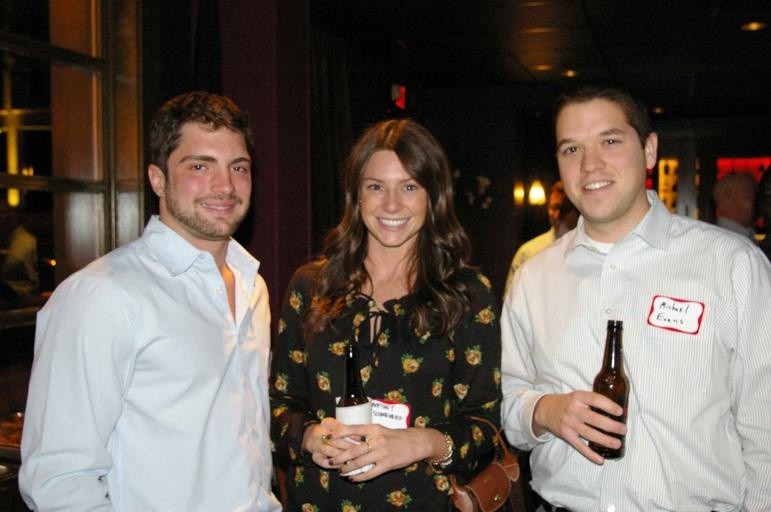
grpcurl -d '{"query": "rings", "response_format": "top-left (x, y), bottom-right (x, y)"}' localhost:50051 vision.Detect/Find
top-left (365, 441), bottom-right (372, 453)
top-left (321, 435), bottom-right (326, 443)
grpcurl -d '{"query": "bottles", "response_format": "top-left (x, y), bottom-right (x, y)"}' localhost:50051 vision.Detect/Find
top-left (337, 344), bottom-right (374, 443)
top-left (588, 319), bottom-right (631, 460)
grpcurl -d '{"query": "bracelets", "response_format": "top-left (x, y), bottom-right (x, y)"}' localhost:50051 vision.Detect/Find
top-left (427, 431), bottom-right (454, 464)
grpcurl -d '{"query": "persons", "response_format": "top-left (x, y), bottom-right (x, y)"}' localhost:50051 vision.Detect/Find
top-left (503, 182), bottom-right (579, 297)
top-left (18, 90), bottom-right (284, 512)
top-left (499, 85), bottom-right (770, 512)
top-left (1, 207), bottom-right (43, 311)
top-left (267, 118), bottom-right (521, 511)
top-left (713, 171), bottom-right (760, 247)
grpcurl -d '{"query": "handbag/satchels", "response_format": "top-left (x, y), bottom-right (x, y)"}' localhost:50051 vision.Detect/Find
top-left (455, 452), bottom-right (520, 512)
